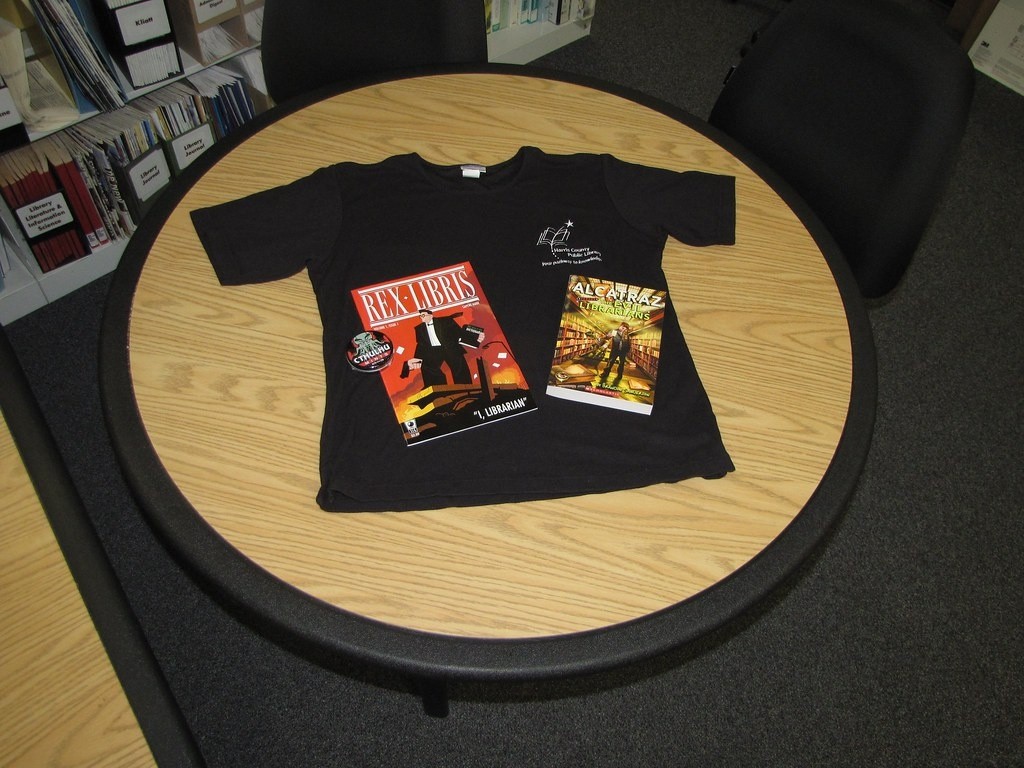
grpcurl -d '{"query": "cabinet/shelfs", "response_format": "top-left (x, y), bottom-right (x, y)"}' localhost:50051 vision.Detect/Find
top-left (0, 0), bottom-right (599, 325)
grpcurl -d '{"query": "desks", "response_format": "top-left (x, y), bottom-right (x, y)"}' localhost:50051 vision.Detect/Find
top-left (0, 335), bottom-right (199, 768)
top-left (100, 62), bottom-right (873, 716)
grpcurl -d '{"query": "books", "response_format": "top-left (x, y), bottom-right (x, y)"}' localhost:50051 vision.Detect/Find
top-left (545, 275), bottom-right (667, 416)
top-left (485, 0), bottom-right (580, 33)
top-left (352, 260), bottom-right (539, 446)
top-left (0, 0), bottom-right (265, 274)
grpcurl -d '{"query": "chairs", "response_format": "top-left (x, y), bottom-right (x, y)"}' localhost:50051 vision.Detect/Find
top-left (262, 0), bottom-right (487, 105)
top-left (707, 0), bottom-right (975, 301)
